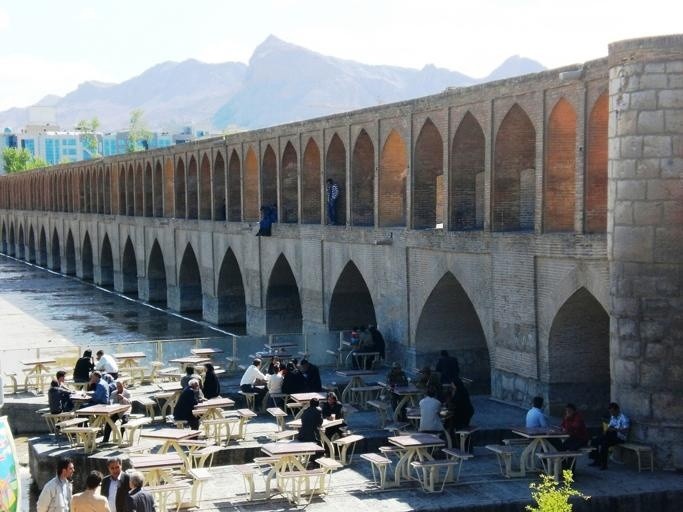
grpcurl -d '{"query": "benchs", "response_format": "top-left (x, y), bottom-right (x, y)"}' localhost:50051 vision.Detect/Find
top-left (0, 342), bottom-right (655, 511)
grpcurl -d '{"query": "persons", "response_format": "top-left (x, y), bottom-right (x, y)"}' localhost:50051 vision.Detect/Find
top-left (387, 350), bottom-right (474, 458)
top-left (525, 394), bottom-right (585, 466)
top-left (73, 348), bottom-right (119, 427)
top-left (101, 457), bottom-right (129, 512)
top-left (70, 470), bottom-right (111, 512)
top-left (172, 362), bottom-right (221, 443)
top-left (588, 401), bottom-right (629, 467)
top-left (49, 370), bottom-right (73, 414)
top-left (126, 471), bottom-right (155, 512)
top-left (262, 204), bottom-right (277, 236)
top-left (345, 325), bottom-right (385, 372)
top-left (297, 391), bottom-right (347, 468)
top-left (99, 381), bottom-right (132, 448)
top-left (240, 355), bottom-right (321, 413)
top-left (326, 179), bottom-right (340, 225)
top-left (37, 460), bottom-right (74, 512)
top-left (255, 206), bottom-right (271, 236)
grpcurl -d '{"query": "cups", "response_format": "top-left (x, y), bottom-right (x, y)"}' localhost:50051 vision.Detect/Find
top-left (330, 414), bottom-right (335, 421)
